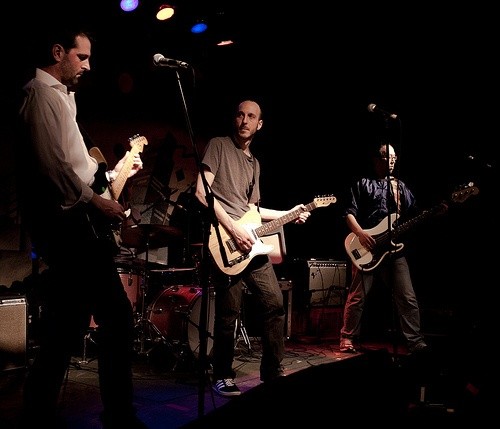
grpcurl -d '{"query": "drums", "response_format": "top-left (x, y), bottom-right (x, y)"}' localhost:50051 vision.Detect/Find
top-left (85, 260), bottom-right (157, 329)
top-left (149, 284), bottom-right (217, 360)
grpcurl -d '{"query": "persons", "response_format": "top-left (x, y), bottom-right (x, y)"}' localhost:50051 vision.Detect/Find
top-left (339, 141), bottom-right (449, 353)
top-left (17, 22), bottom-right (149, 429)
top-left (196, 98), bottom-right (311, 398)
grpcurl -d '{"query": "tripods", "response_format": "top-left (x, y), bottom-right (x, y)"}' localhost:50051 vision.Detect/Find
top-left (130, 242), bottom-right (185, 363)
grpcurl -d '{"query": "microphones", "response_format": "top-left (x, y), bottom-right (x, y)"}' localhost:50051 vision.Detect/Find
top-left (368, 104), bottom-right (397, 119)
top-left (469, 156), bottom-right (492, 168)
top-left (153, 53), bottom-right (188, 68)
top-left (168, 200), bottom-right (187, 211)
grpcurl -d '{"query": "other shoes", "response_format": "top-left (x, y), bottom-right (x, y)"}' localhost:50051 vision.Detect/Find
top-left (339, 336), bottom-right (355, 353)
top-left (405, 341), bottom-right (427, 350)
top-left (209, 375), bottom-right (241, 396)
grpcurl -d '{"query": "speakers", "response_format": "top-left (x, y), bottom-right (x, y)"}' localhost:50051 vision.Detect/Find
top-left (0, 294), bottom-right (28, 371)
top-left (183, 347), bottom-right (409, 429)
top-left (305, 260), bottom-right (347, 307)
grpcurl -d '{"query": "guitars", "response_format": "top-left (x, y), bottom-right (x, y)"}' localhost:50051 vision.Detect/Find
top-left (344, 182), bottom-right (480, 272)
top-left (65, 134), bottom-right (149, 258)
top-left (205, 194), bottom-right (337, 277)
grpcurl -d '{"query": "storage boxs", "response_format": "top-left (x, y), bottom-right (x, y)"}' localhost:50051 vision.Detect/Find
top-left (242, 280), bottom-right (294, 343)
